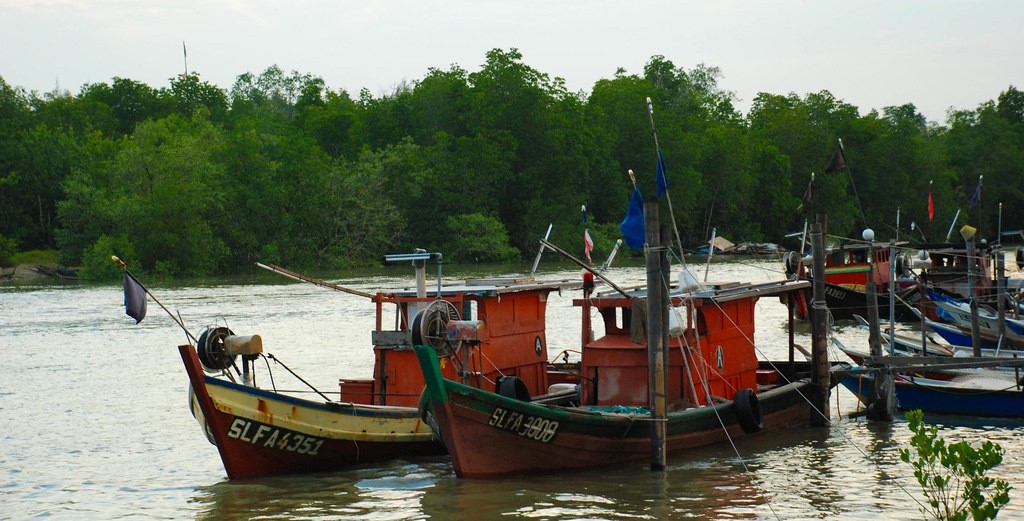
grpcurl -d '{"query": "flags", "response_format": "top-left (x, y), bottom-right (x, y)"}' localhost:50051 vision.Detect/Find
top-left (582, 208), bottom-right (587, 223)
top-left (655, 151), bottom-right (667, 199)
top-left (799, 183), bottom-right (813, 209)
top-left (823, 147), bottom-right (847, 174)
top-left (928, 193), bottom-right (935, 221)
top-left (970, 186), bottom-right (980, 210)
top-left (584, 229), bottom-right (594, 262)
top-left (620, 186), bottom-right (645, 250)
top-left (121, 269), bottom-right (148, 324)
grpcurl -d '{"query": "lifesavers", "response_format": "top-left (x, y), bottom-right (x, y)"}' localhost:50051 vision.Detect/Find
top-left (734, 388), bottom-right (764, 434)
top-left (500, 376), bottom-right (530, 402)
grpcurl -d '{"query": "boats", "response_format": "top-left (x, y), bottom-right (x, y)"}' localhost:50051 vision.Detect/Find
top-left (417, 91), bottom-right (851, 486)
top-left (778, 135), bottom-right (1024, 422)
top-left (109, 249), bottom-right (610, 483)
